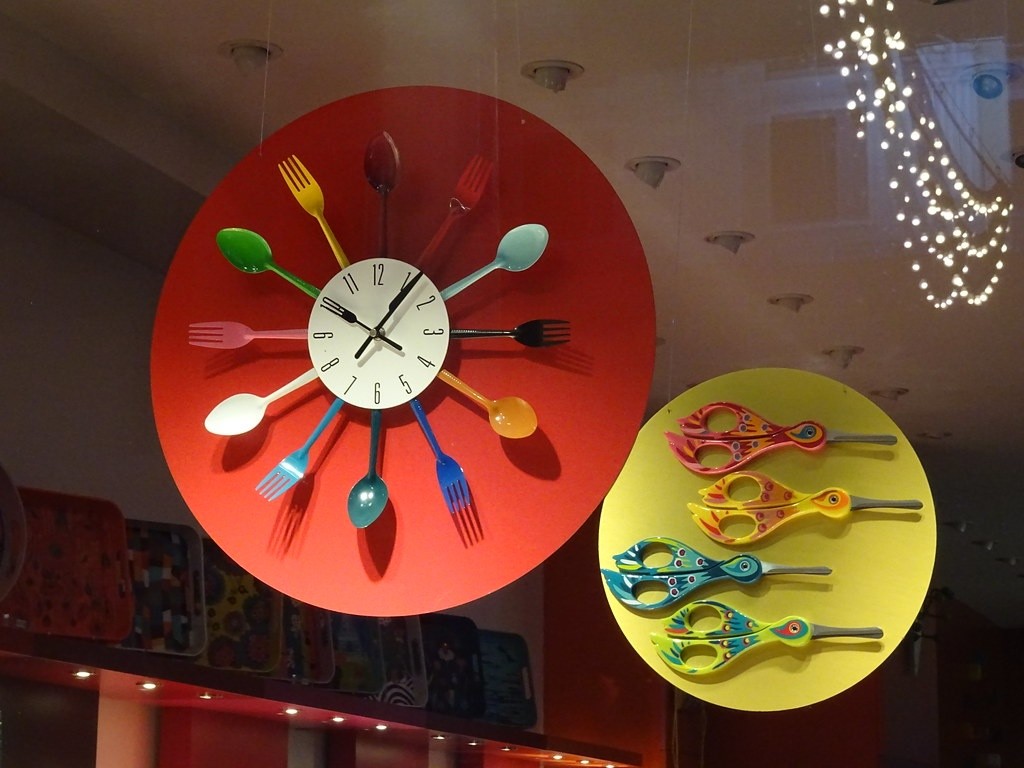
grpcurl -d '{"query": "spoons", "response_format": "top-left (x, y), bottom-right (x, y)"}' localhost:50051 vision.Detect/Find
top-left (347, 409), bottom-right (388, 528)
top-left (439, 224), bottom-right (548, 301)
top-left (437, 368), bottom-right (537, 439)
top-left (364, 131), bottom-right (400, 257)
top-left (216, 227), bottom-right (321, 299)
top-left (205, 367), bottom-right (319, 436)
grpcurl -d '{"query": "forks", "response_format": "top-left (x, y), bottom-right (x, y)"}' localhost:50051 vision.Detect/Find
top-left (255, 394), bottom-right (344, 502)
top-left (449, 319), bottom-right (570, 347)
top-left (277, 155), bottom-right (350, 269)
top-left (189, 321), bottom-right (308, 348)
top-left (414, 153), bottom-right (493, 270)
top-left (408, 398), bottom-right (470, 514)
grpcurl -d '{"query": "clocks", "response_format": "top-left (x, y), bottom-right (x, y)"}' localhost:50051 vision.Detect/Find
top-left (306, 260), bottom-right (454, 413)
